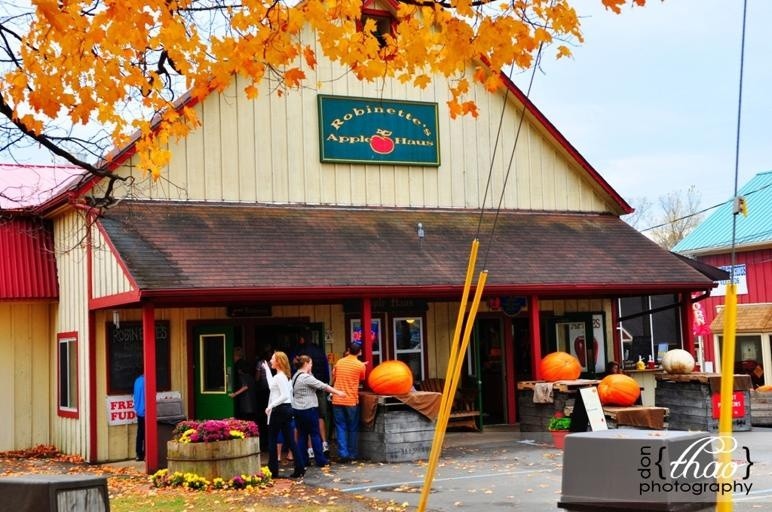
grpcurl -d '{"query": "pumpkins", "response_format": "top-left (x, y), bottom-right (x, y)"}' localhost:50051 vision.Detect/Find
top-left (368, 361), bottom-right (412, 394)
top-left (598, 374), bottom-right (640, 405)
top-left (540, 352), bottom-right (580, 381)
top-left (757, 385), bottom-right (772, 392)
top-left (663, 349), bottom-right (694, 374)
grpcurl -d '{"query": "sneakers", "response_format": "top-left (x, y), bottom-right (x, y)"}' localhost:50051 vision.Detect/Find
top-left (289, 446), bottom-right (349, 479)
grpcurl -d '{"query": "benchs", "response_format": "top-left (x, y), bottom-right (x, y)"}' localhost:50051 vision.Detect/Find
top-left (413, 378), bottom-right (480, 432)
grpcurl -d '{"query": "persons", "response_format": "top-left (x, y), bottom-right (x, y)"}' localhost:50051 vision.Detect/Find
top-left (228, 329), bottom-right (373, 478)
top-left (599, 361), bottom-right (624, 378)
top-left (134, 372), bottom-right (145, 462)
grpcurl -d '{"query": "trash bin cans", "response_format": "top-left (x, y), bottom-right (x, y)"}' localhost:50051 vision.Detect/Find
top-left (157, 398), bottom-right (186, 469)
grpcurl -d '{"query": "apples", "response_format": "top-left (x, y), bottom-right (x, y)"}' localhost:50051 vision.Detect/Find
top-left (574, 335), bottom-right (598, 367)
top-left (370, 135), bottom-right (394, 153)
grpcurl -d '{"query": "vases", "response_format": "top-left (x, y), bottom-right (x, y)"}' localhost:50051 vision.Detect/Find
top-left (550, 430), bottom-right (569, 449)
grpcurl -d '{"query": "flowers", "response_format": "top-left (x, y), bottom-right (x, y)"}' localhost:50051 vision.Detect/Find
top-left (172, 415), bottom-right (260, 446)
top-left (151, 464), bottom-right (273, 491)
top-left (544, 412), bottom-right (571, 431)
